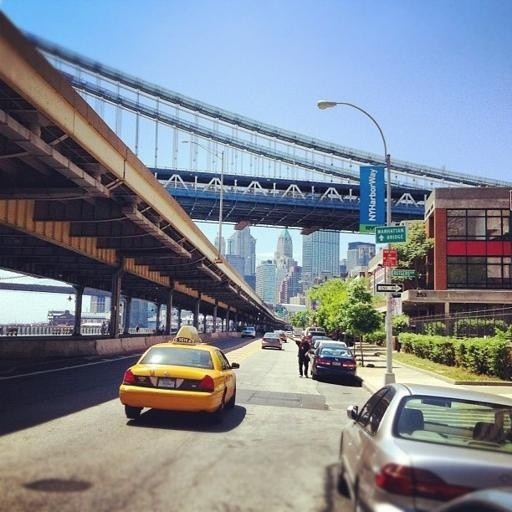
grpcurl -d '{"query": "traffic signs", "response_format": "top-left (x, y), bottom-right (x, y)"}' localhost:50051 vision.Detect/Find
top-left (382, 247), bottom-right (397, 268)
top-left (375, 281), bottom-right (404, 293)
top-left (374, 226), bottom-right (406, 242)
top-left (390, 268), bottom-right (417, 278)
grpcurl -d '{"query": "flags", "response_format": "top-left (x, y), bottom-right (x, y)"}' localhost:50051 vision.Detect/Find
top-left (359, 165), bottom-right (386, 233)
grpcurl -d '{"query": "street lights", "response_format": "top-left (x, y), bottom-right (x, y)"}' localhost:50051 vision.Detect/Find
top-left (179, 138), bottom-right (225, 257)
top-left (317, 101), bottom-right (398, 388)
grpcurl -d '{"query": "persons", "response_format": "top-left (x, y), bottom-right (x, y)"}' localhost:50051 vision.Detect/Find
top-left (284, 333), bottom-right (310, 378)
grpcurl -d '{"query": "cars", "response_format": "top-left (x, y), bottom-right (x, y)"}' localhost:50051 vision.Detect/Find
top-left (275, 325), bottom-right (347, 360)
top-left (428, 488), bottom-right (512, 510)
top-left (309, 345), bottom-right (357, 384)
top-left (241, 327), bottom-right (256, 339)
top-left (336, 380), bottom-right (512, 511)
top-left (118, 325), bottom-right (240, 424)
top-left (261, 332), bottom-right (282, 350)
top-left (168, 326), bottom-right (178, 334)
